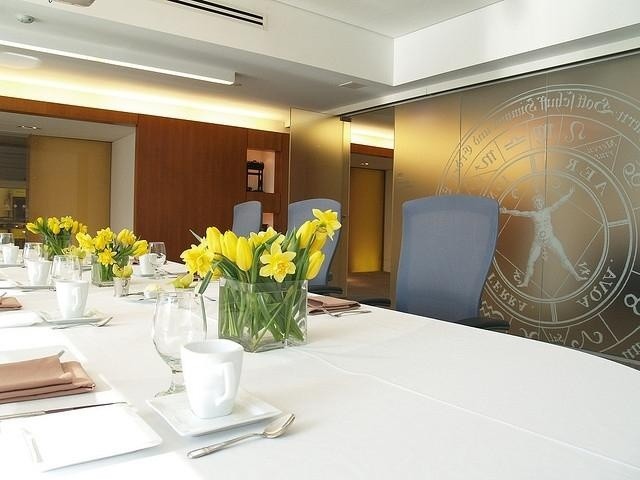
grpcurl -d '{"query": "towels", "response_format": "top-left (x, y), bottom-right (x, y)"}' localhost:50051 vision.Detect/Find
top-left (308, 297), bottom-right (360, 315)
top-left (0, 356), bottom-right (96, 404)
top-left (0, 297), bottom-right (22, 311)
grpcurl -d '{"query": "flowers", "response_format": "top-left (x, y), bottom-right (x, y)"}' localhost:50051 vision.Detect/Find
top-left (174, 209), bottom-right (341, 347)
top-left (26, 217), bottom-right (86, 260)
top-left (71, 229), bottom-right (146, 282)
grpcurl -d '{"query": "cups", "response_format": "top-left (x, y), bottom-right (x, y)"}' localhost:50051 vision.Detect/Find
top-left (179, 338), bottom-right (244, 420)
top-left (55, 281), bottom-right (91, 316)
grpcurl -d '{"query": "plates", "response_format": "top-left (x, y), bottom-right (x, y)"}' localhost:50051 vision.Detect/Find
top-left (41, 311), bottom-right (102, 324)
top-left (146, 389), bottom-right (282, 437)
top-left (23, 417), bottom-right (162, 471)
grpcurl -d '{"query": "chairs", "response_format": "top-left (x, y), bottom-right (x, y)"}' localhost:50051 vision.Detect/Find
top-left (357, 194), bottom-right (510, 331)
top-left (232, 201), bottom-right (262, 240)
top-left (287, 198), bottom-right (343, 296)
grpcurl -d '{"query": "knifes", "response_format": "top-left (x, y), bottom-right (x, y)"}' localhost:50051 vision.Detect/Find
top-left (0, 400), bottom-right (124, 419)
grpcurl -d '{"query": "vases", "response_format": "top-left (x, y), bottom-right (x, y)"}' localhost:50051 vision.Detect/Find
top-left (91, 255), bottom-right (126, 287)
top-left (43, 240), bottom-right (68, 260)
top-left (219, 277), bottom-right (308, 353)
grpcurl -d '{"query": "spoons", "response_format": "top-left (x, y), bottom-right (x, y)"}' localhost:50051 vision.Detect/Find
top-left (54, 317), bottom-right (113, 329)
top-left (189, 412), bottom-right (295, 460)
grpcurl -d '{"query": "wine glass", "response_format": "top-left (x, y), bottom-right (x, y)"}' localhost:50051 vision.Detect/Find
top-left (153, 291), bottom-right (206, 401)
top-left (0, 233), bottom-right (15, 261)
top-left (0, 240), bottom-right (193, 301)
